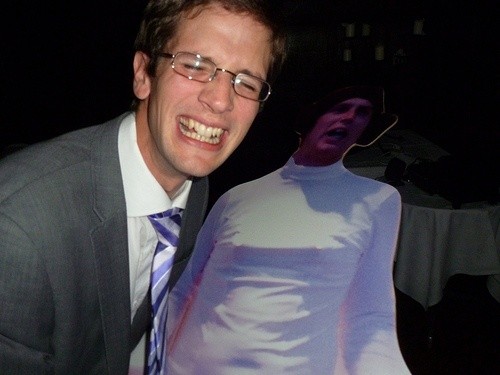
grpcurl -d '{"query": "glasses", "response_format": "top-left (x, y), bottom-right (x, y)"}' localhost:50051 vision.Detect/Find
top-left (156, 51), bottom-right (271, 102)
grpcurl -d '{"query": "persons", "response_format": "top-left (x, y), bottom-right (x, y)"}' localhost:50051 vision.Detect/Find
top-left (160, 79), bottom-right (413, 375)
top-left (374, 154), bottom-right (454, 207)
top-left (0, 0), bottom-right (290, 375)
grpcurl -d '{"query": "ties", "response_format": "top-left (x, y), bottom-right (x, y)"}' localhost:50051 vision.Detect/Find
top-left (147, 207), bottom-right (184, 375)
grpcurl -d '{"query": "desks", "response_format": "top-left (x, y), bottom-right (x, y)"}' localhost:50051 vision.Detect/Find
top-left (393, 183), bottom-right (500, 311)
